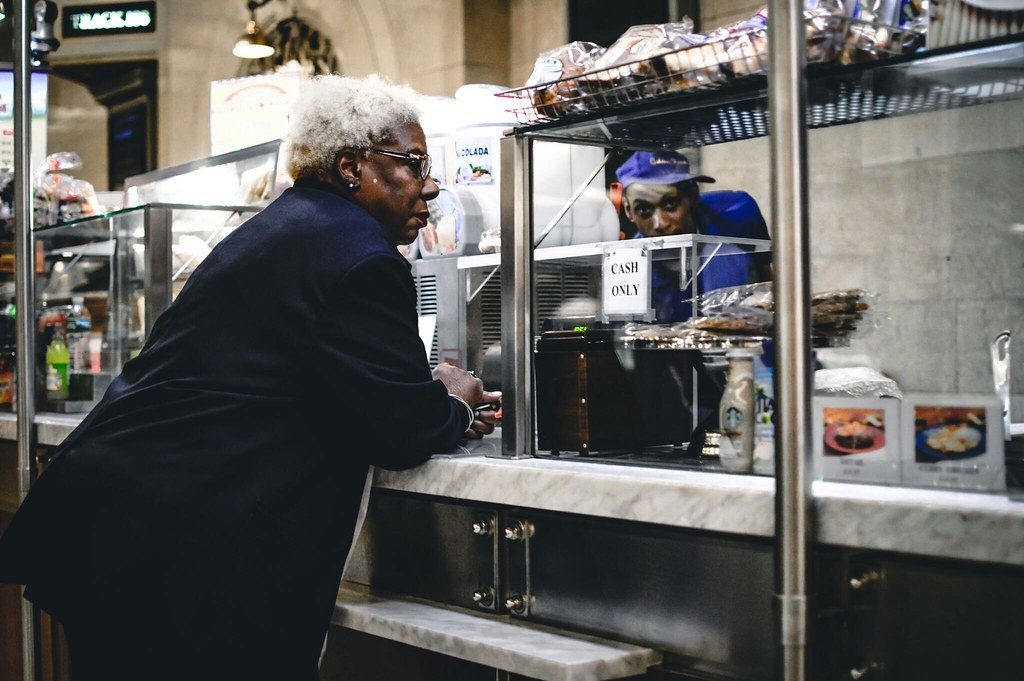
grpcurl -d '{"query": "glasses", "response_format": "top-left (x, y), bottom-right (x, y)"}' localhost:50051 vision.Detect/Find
top-left (331, 144), bottom-right (431, 181)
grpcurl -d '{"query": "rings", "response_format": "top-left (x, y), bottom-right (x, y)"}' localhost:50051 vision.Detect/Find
top-left (468, 371), bottom-right (478, 378)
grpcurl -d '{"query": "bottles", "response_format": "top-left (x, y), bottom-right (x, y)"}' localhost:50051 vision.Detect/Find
top-left (44, 323), bottom-right (70, 399)
top-left (753, 367), bottom-right (775, 476)
top-left (0, 309), bottom-right (16, 412)
top-left (718, 349), bottom-right (757, 474)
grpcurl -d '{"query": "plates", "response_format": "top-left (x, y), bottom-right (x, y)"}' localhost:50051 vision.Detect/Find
top-left (914, 420), bottom-right (987, 459)
top-left (823, 422), bottom-right (886, 455)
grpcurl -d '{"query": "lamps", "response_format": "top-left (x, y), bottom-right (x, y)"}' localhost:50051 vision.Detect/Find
top-left (233, 0), bottom-right (275, 58)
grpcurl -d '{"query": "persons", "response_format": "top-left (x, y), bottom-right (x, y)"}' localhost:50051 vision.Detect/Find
top-left (615, 146), bottom-right (783, 366)
top-left (0, 73), bottom-right (502, 681)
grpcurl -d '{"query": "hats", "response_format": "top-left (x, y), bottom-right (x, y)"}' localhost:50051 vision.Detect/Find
top-left (614, 147), bottom-right (716, 190)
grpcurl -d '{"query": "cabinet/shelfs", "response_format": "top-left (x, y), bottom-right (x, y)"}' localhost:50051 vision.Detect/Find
top-left (38, 199), bottom-right (270, 418)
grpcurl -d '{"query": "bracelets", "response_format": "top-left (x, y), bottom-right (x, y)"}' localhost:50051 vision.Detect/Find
top-left (447, 392), bottom-right (475, 432)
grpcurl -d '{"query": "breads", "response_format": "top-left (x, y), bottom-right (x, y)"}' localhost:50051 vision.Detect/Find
top-left (530, 0), bottom-right (924, 118)
